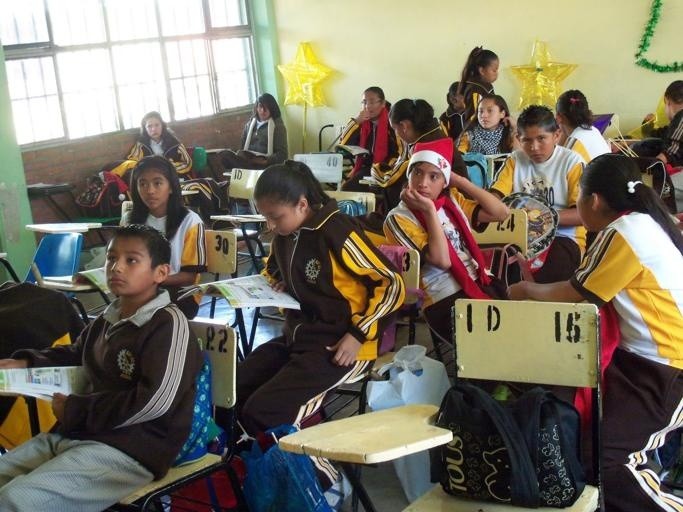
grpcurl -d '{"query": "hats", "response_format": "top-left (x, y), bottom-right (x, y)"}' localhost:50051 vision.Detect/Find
top-left (405, 135), bottom-right (455, 190)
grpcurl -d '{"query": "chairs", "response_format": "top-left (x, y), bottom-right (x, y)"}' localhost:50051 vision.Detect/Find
top-left (0, 146), bottom-right (528, 512)
top-left (279, 299), bottom-right (603, 511)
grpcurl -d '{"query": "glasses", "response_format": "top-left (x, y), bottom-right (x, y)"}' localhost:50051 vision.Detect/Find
top-left (361, 100), bottom-right (382, 105)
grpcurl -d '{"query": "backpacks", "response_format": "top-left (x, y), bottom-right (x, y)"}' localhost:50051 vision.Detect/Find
top-left (173, 344), bottom-right (215, 468)
top-left (499, 192), bottom-right (560, 274)
top-left (461, 150), bottom-right (490, 191)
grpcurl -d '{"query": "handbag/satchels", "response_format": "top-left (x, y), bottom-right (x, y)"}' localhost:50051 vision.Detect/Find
top-left (437, 380), bottom-right (586, 509)
top-left (240, 425), bottom-right (333, 512)
top-left (356, 345), bottom-right (451, 499)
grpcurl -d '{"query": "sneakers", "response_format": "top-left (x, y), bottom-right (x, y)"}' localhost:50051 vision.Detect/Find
top-left (326, 473), bottom-right (354, 507)
